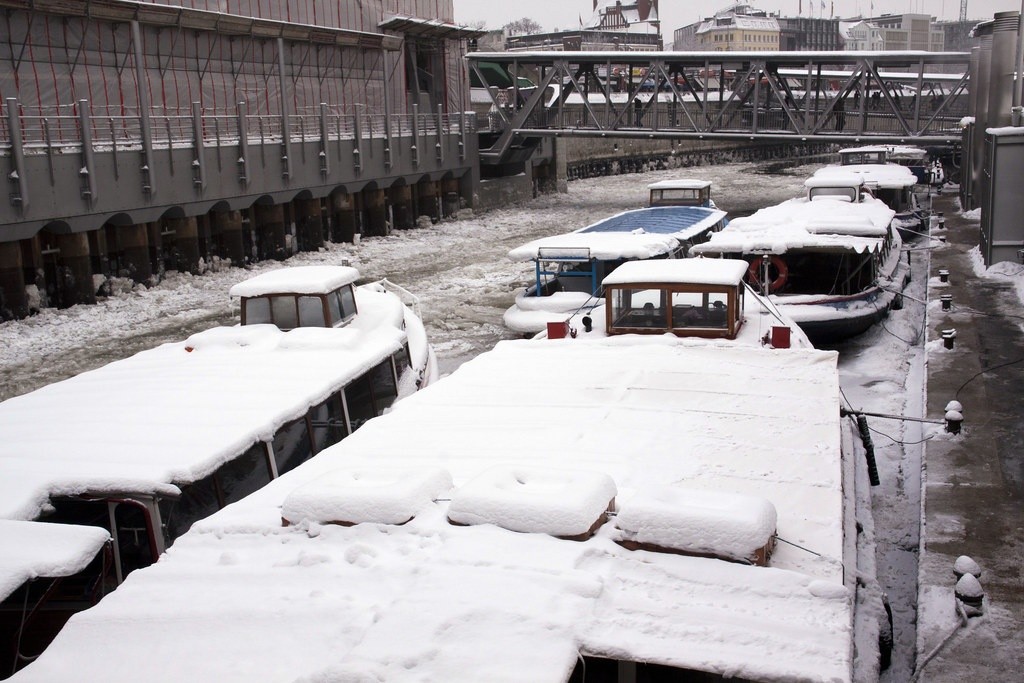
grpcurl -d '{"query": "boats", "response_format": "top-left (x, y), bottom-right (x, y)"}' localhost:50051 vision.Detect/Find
top-left (502, 178), bottom-right (729, 334)
top-left (860, 141), bottom-right (930, 184)
top-left (811, 146), bottom-right (922, 240)
top-left (687, 173), bottom-right (911, 338)
top-left (0, 268), bottom-right (439, 683)
top-left (3, 259), bottom-right (895, 683)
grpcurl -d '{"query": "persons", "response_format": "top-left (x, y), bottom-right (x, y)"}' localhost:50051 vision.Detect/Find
top-left (781, 96), bottom-right (790, 131)
top-left (853, 90), bottom-right (945, 113)
top-left (833, 96), bottom-right (847, 132)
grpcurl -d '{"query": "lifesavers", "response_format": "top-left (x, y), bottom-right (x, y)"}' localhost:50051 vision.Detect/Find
top-left (747, 254), bottom-right (790, 293)
